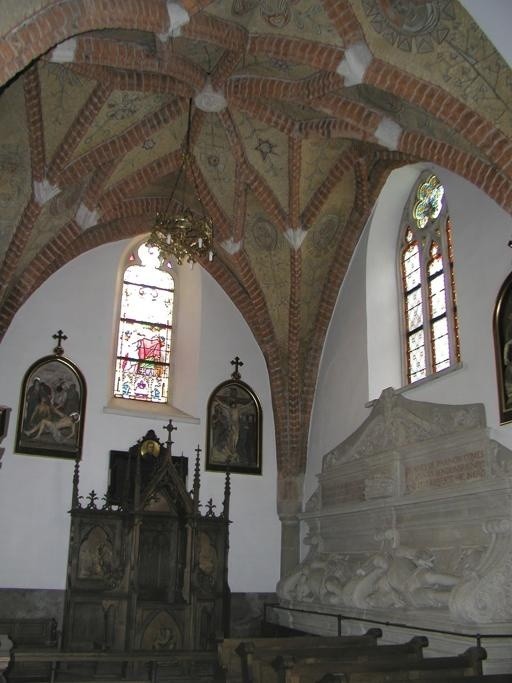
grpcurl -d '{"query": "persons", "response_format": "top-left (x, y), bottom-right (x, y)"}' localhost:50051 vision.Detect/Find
top-left (216, 398), bottom-right (254, 450)
top-left (22, 376), bottom-right (80, 442)
top-left (142, 440), bottom-right (157, 458)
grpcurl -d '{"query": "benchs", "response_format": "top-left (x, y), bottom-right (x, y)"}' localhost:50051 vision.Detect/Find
top-left (0, 618), bottom-right (58, 680)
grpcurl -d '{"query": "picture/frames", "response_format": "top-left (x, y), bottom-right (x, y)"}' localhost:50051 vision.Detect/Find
top-left (204, 379), bottom-right (263, 476)
top-left (13, 354), bottom-right (87, 462)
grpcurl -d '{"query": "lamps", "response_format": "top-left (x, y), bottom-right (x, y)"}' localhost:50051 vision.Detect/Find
top-left (145, 96), bottom-right (216, 269)
